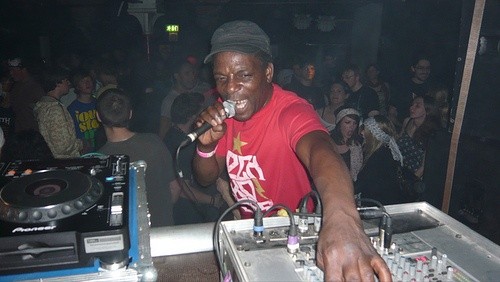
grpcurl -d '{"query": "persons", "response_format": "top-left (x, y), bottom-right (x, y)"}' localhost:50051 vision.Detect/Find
top-left (398, 94), bottom-right (438, 207)
top-left (0, 59), bottom-right (46, 160)
top-left (215, 171), bottom-right (241, 225)
top-left (95, 89), bottom-right (182, 228)
top-left (67, 68), bottom-right (107, 155)
top-left (360, 115), bottom-right (405, 206)
top-left (91, 54), bottom-right (213, 139)
top-left (192, 20), bottom-right (393, 282)
top-left (271, 47), bottom-right (448, 133)
top-left (163, 91), bottom-right (225, 224)
top-left (32, 70), bottom-right (92, 158)
top-left (328, 104), bottom-right (364, 183)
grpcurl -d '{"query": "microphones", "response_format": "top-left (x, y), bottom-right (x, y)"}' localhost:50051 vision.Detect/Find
top-left (181, 99), bottom-right (237, 148)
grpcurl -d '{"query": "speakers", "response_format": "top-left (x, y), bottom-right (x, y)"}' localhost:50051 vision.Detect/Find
top-left (446, 0), bottom-right (500, 142)
top-left (420, 128), bottom-right (500, 246)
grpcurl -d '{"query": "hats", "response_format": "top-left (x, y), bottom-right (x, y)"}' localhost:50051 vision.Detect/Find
top-left (201, 19), bottom-right (273, 63)
top-left (334, 104), bottom-right (361, 124)
